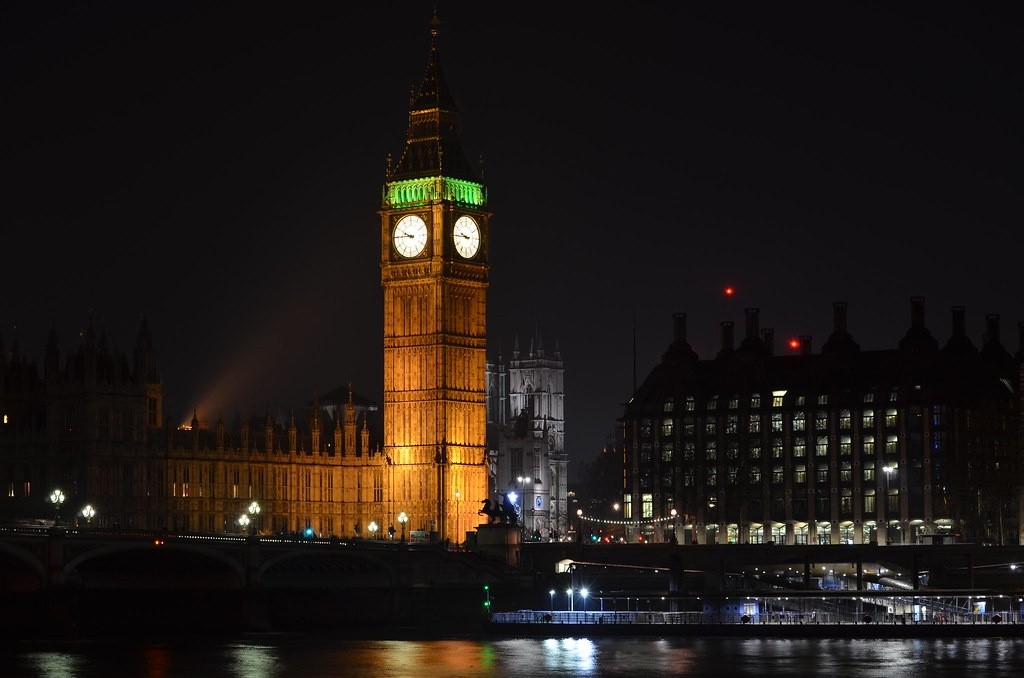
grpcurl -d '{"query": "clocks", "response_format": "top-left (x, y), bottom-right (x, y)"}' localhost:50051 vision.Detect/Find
top-left (389, 211), bottom-right (433, 261)
top-left (449, 211), bottom-right (484, 265)
